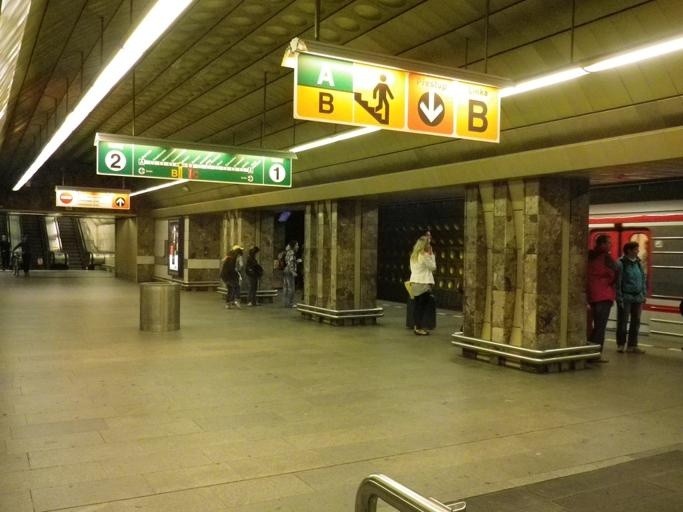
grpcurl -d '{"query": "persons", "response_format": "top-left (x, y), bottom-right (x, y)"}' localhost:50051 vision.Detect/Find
top-left (0, 234), bottom-right (10, 271)
top-left (13, 234), bottom-right (31, 277)
top-left (246, 247), bottom-right (264, 306)
top-left (219, 246), bottom-right (244, 309)
top-left (172, 225), bottom-right (178, 264)
top-left (406, 230), bottom-right (432, 329)
top-left (410, 236), bottom-right (437, 336)
top-left (587, 236), bottom-right (621, 363)
top-left (616, 242), bottom-right (647, 354)
top-left (283, 240), bottom-right (302, 308)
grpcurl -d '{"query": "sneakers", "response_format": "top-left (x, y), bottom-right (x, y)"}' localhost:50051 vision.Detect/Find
top-left (616, 345), bottom-right (623, 352)
top-left (625, 346), bottom-right (644, 353)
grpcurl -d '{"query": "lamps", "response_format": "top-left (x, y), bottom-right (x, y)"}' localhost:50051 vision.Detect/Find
top-left (11, 0), bottom-right (192, 198)
top-left (279, 1), bottom-right (683, 154)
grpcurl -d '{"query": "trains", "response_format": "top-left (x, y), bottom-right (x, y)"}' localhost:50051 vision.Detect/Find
top-left (587, 197), bottom-right (682, 337)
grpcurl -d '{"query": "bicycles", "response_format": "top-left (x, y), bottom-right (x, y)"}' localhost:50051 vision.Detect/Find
top-left (8, 250), bottom-right (24, 278)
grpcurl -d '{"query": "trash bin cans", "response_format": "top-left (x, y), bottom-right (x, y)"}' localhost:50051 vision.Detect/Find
top-left (140, 282), bottom-right (180, 333)
